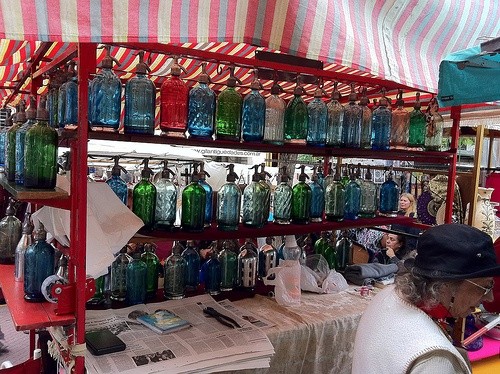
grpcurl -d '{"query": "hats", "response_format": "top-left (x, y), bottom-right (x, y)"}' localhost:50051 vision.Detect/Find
top-left (403, 224), bottom-right (500, 280)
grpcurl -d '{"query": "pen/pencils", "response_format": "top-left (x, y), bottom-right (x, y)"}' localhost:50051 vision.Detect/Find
top-left (176, 315), bottom-right (193, 328)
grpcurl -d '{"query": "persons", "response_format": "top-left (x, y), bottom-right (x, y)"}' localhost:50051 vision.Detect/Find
top-left (391, 193), bottom-right (419, 252)
top-left (351, 223), bottom-right (500, 374)
top-left (372, 229), bottom-right (418, 277)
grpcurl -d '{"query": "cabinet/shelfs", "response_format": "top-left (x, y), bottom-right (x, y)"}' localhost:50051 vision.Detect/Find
top-left (2, 37), bottom-right (462, 374)
top-left (443, 125), bottom-right (500, 230)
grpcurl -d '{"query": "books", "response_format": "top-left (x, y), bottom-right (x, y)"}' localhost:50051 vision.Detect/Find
top-left (136, 310), bottom-right (191, 336)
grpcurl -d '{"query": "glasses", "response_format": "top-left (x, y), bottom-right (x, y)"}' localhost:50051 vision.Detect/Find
top-left (464, 279), bottom-right (500, 296)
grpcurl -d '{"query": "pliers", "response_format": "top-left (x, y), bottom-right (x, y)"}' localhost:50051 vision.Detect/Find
top-left (202, 306), bottom-right (241, 330)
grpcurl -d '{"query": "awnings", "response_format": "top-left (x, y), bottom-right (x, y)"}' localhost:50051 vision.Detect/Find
top-left (186, 146), bottom-right (266, 158)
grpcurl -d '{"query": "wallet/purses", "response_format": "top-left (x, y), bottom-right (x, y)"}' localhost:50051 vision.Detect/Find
top-left (85, 330), bottom-right (127, 355)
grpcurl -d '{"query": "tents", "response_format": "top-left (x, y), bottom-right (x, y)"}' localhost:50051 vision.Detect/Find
top-left (0, 0), bottom-right (499, 374)
top-left (436, 36), bottom-right (500, 224)
top-left (58, 139), bottom-right (208, 177)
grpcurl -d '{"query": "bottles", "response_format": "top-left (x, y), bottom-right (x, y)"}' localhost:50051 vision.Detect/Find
top-left (0, 44), bottom-right (444, 306)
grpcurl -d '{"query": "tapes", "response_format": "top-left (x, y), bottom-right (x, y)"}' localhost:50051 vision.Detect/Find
top-left (41, 274), bottom-right (70, 303)
